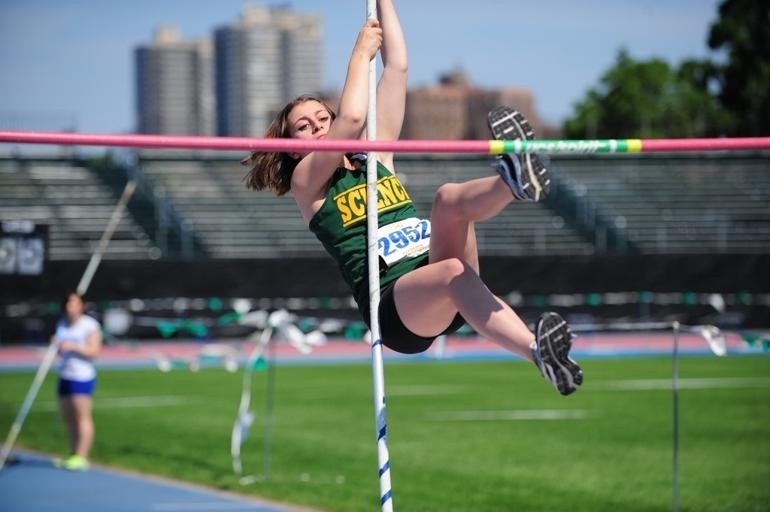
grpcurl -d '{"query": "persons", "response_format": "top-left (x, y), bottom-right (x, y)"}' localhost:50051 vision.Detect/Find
top-left (240, 0), bottom-right (588, 400)
top-left (48, 290), bottom-right (104, 472)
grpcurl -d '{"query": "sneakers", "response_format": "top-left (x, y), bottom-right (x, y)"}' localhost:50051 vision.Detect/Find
top-left (52, 453), bottom-right (91, 471)
top-left (531, 310), bottom-right (584, 396)
top-left (486, 105), bottom-right (552, 204)
